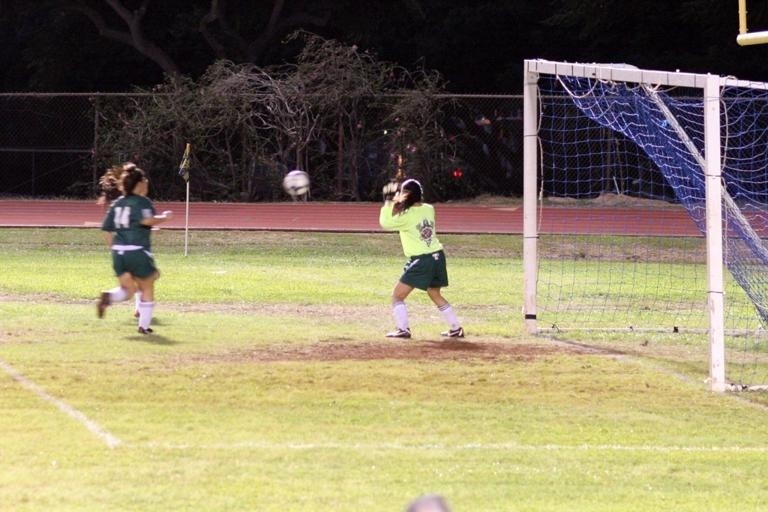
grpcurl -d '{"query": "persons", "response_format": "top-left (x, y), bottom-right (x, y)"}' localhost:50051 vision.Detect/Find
top-left (379, 179), bottom-right (464, 337)
top-left (98, 162), bottom-right (174, 334)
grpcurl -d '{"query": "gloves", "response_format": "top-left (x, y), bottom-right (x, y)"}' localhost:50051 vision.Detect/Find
top-left (383, 182), bottom-right (399, 202)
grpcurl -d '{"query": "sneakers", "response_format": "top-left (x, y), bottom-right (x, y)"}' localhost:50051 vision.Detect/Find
top-left (440, 326), bottom-right (464, 337)
top-left (385, 326), bottom-right (411, 338)
top-left (97, 292), bottom-right (112, 319)
top-left (133, 310), bottom-right (153, 334)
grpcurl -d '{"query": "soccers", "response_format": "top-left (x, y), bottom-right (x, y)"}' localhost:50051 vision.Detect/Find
top-left (284, 171), bottom-right (309, 197)
